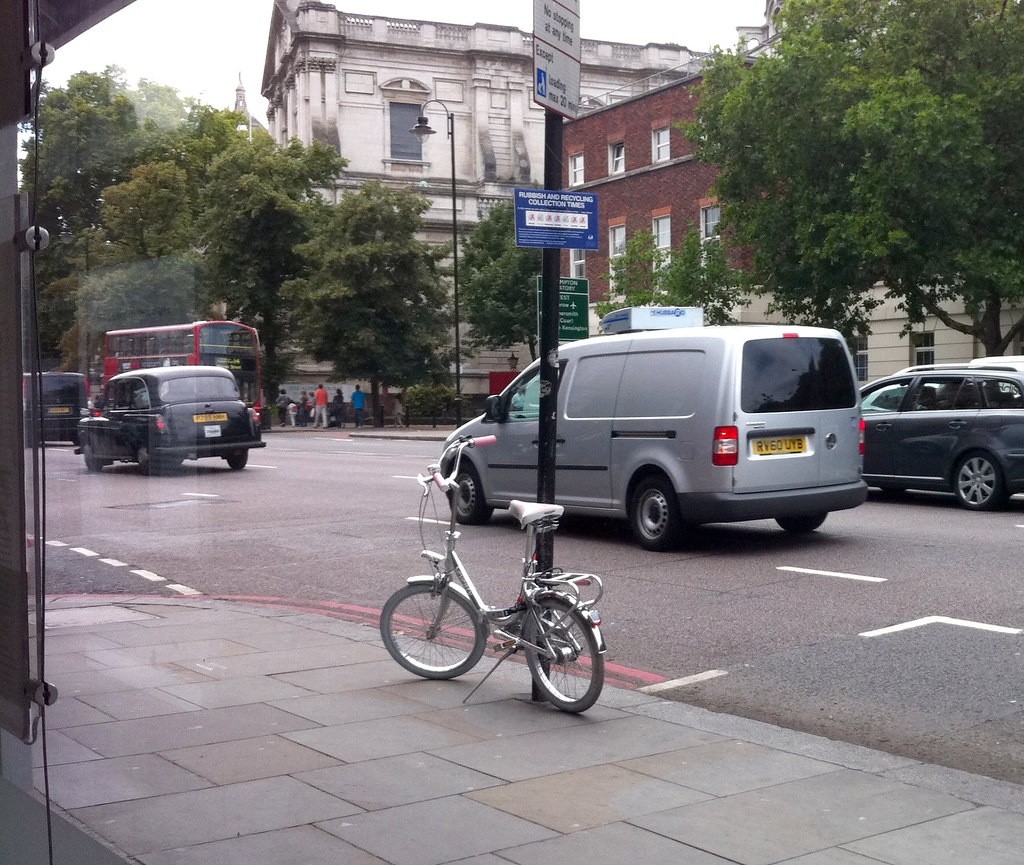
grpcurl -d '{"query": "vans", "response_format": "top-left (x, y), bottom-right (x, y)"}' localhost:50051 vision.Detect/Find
top-left (440, 306), bottom-right (869, 551)
top-left (23, 371), bottom-right (89, 449)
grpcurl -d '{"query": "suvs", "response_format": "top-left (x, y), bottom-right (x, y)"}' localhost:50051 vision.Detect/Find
top-left (857, 364), bottom-right (1024, 514)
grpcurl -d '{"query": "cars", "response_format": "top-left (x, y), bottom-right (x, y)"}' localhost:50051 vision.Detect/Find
top-left (75, 366), bottom-right (265, 476)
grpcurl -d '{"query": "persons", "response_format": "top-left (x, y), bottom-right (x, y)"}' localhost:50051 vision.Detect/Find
top-left (312, 384), bottom-right (328, 428)
top-left (306, 392), bottom-right (320, 427)
top-left (287, 400), bottom-right (303, 426)
top-left (332, 389), bottom-right (345, 428)
top-left (277, 389), bottom-right (288, 427)
top-left (392, 393), bottom-right (404, 427)
top-left (349, 384), bottom-right (367, 428)
top-left (298, 391), bottom-right (308, 426)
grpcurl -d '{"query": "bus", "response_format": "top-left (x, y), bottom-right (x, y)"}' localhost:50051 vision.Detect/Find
top-left (100, 319), bottom-right (261, 414)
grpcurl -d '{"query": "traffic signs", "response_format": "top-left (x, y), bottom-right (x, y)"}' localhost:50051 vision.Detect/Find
top-left (536, 275), bottom-right (589, 342)
top-left (532, 0), bottom-right (581, 122)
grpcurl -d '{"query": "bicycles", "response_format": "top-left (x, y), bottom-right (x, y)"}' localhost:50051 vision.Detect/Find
top-left (379, 433), bottom-right (608, 714)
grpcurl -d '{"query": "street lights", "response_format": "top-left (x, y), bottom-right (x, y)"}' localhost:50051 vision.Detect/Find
top-left (408, 98), bottom-right (463, 432)
